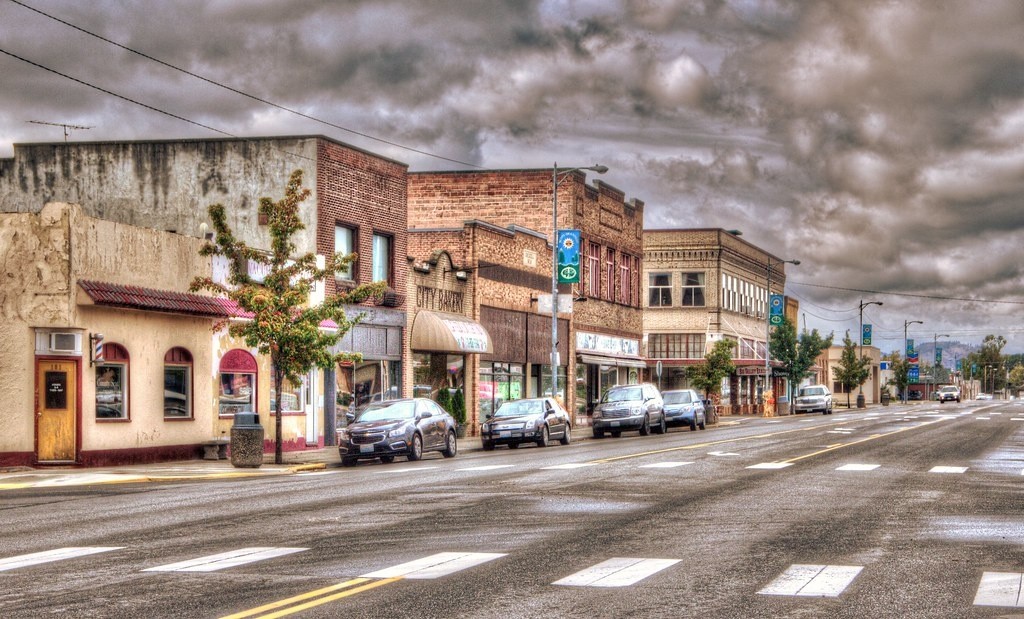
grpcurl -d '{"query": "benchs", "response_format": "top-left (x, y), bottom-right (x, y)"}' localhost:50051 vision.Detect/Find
top-left (199, 438), bottom-right (231, 460)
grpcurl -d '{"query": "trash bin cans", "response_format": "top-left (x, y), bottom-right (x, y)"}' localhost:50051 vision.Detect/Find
top-left (777, 396), bottom-right (790, 416)
top-left (857, 394), bottom-right (865, 408)
top-left (702, 399), bottom-right (714, 424)
top-left (231, 412), bottom-right (264, 468)
top-left (882, 394), bottom-right (890, 406)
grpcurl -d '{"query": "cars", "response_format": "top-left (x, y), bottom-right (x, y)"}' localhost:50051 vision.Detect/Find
top-left (794, 385), bottom-right (833, 415)
top-left (908, 390), bottom-right (923, 400)
top-left (660, 389), bottom-right (705, 431)
top-left (976, 393), bottom-right (985, 400)
top-left (338, 397), bottom-right (460, 467)
top-left (480, 398), bottom-right (572, 450)
top-left (939, 386), bottom-right (961, 403)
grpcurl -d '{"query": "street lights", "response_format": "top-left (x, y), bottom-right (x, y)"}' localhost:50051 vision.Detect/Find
top-left (857, 299), bottom-right (884, 407)
top-left (932, 332), bottom-right (951, 400)
top-left (985, 366), bottom-right (992, 394)
top-left (549, 160), bottom-right (610, 396)
top-left (993, 369), bottom-right (997, 395)
top-left (903, 320), bottom-right (923, 404)
top-left (766, 257), bottom-right (802, 391)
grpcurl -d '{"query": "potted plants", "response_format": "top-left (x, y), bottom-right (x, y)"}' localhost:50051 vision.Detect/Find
top-left (451, 386), bottom-right (471, 438)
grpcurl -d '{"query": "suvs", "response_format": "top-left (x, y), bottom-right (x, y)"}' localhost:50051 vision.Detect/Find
top-left (592, 383), bottom-right (666, 438)
